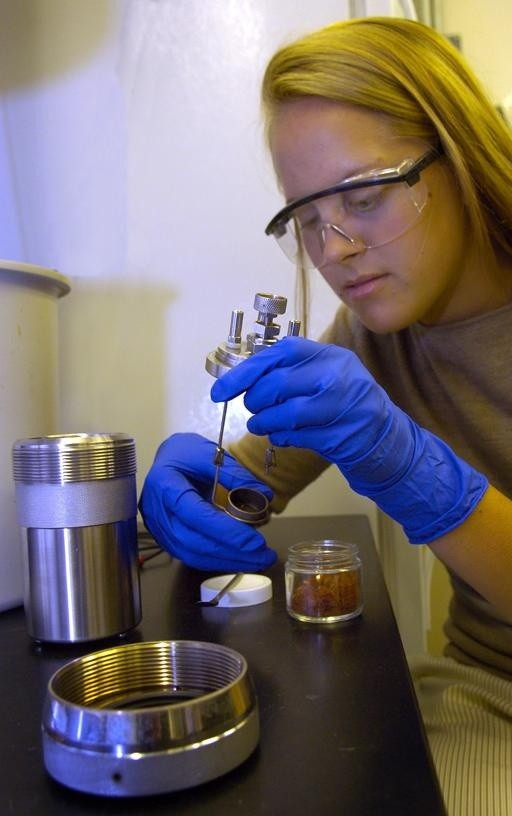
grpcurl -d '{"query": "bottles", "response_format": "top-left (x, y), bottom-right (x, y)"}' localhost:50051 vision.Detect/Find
top-left (283, 539), bottom-right (363, 624)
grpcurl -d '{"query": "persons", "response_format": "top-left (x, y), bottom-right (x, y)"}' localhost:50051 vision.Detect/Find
top-left (138, 15), bottom-right (512, 816)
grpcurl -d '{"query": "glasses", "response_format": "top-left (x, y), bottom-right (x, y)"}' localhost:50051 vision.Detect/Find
top-left (265, 148), bottom-right (445, 270)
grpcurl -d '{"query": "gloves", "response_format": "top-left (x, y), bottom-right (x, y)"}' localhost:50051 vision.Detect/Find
top-left (210, 338), bottom-right (489, 544)
top-left (136, 431), bottom-right (277, 575)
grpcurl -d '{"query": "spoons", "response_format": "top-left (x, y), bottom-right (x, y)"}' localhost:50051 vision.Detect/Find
top-left (195, 570), bottom-right (243, 608)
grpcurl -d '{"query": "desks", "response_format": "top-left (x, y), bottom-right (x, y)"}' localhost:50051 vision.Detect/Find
top-left (0, 514), bottom-right (449, 815)
